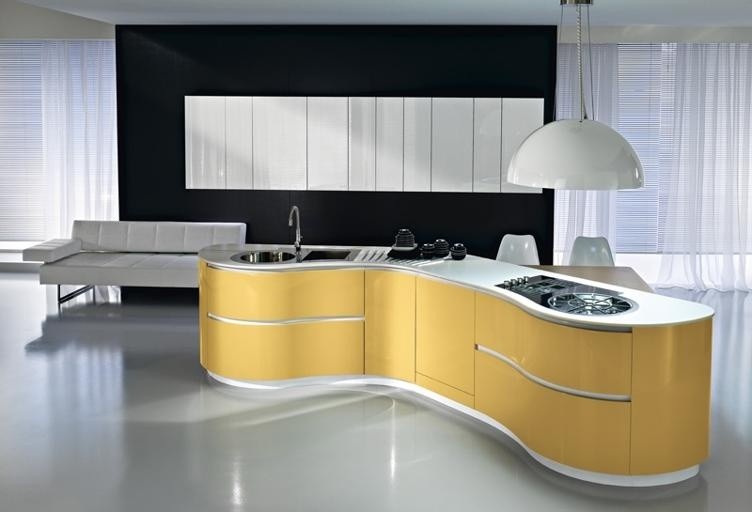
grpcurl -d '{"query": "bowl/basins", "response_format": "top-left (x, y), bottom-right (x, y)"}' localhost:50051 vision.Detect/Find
top-left (422, 238), bottom-right (466, 260)
top-left (395, 229), bottom-right (413, 247)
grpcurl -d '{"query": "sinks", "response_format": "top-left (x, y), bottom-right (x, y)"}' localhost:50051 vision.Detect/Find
top-left (231, 249), bottom-right (301, 264)
top-left (302, 248), bottom-right (362, 262)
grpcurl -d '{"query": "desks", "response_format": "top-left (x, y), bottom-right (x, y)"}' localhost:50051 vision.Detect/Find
top-left (519, 265), bottom-right (656, 296)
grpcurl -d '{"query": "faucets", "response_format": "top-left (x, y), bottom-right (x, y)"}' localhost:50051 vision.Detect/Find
top-left (288, 205), bottom-right (304, 263)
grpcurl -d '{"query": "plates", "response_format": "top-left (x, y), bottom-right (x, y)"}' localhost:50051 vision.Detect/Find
top-left (387, 243), bottom-right (421, 259)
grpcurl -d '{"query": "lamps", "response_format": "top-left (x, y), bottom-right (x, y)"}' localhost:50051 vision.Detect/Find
top-left (506, 0), bottom-right (645, 190)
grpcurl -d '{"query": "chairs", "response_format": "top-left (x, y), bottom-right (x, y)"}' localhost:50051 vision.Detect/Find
top-left (495, 234), bottom-right (540, 266)
top-left (569, 236), bottom-right (614, 267)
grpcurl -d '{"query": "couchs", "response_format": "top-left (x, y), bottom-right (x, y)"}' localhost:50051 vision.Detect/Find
top-left (23, 221), bottom-right (247, 308)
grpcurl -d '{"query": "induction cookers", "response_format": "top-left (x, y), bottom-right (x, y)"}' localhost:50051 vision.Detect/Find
top-left (493, 274), bottom-right (639, 316)
